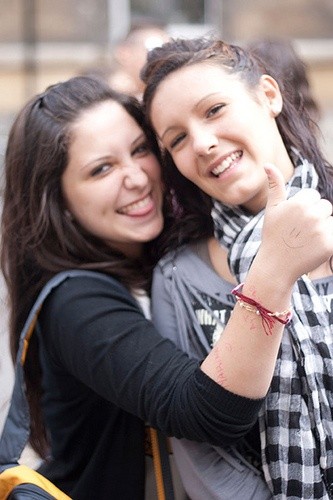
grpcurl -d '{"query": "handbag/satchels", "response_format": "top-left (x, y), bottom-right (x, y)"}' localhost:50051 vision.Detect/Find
top-left (0, 459), bottom-right (74, 500)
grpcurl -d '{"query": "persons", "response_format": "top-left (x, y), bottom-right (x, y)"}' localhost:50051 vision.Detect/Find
top-left (1, 73), bottom-right (333, 500)
top-left (104, 19), bottom-right (322, 135)
top-left (127, 32), bottom-right (333, 500)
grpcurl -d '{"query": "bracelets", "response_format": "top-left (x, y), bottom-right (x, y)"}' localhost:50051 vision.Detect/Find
top-left (230, 282), bottom-right (293, 336)
